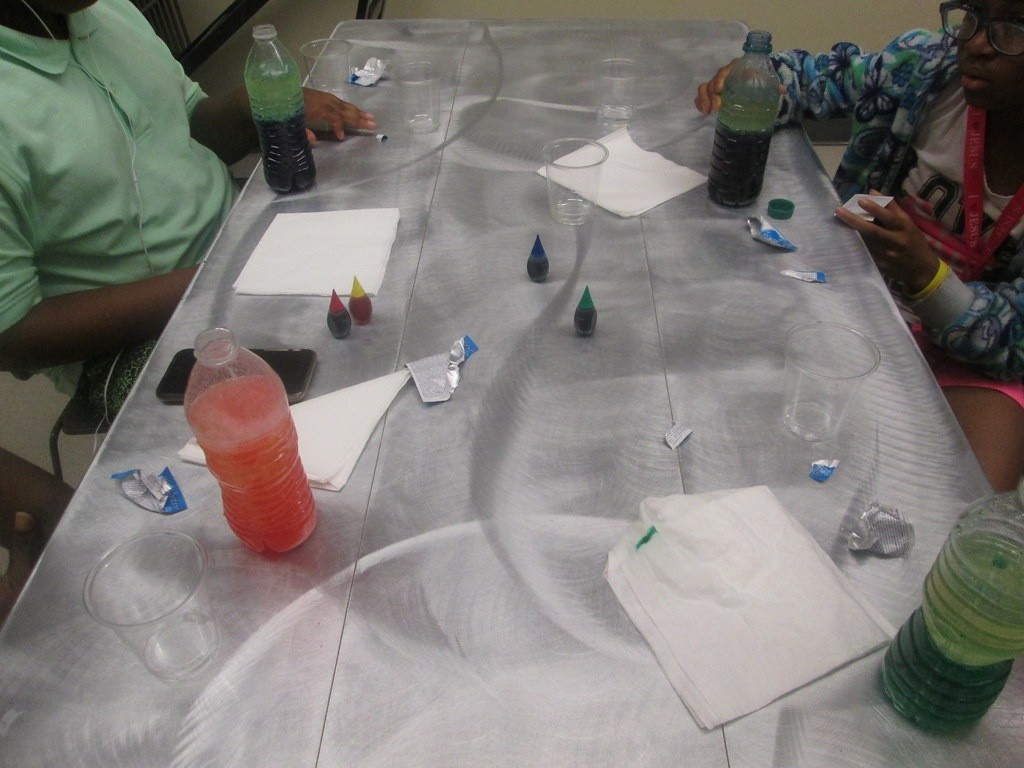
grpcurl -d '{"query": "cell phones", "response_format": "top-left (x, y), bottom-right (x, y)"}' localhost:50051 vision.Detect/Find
top-left (154, 346), bottom-right (316, 407)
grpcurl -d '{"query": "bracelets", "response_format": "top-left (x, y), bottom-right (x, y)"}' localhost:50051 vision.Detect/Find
top-left (902, 257), bottom-right (949, 302)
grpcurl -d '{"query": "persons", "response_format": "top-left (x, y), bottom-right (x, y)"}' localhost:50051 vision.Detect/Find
top-left (694, 0), bottom-right (1024, 500)
top-left (0, 0), bottom-right (374, 425)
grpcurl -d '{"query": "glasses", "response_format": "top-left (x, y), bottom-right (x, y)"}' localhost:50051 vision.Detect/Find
top-left (940, 0), bottom-right (1024, 56)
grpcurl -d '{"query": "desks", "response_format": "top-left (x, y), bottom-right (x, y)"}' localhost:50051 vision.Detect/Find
top-left (0, 21), bottom-right (1024, 768)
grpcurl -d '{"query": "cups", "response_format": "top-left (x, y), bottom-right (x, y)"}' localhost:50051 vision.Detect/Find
top-left (780, 321), bottom-right (880, 441)
top-left (300, 39), bottom-right (352, 102)
top-left (593, 58), bottom-right (641, 128)
top-left (393, 61), bottom-right (440, 133)
top-left (542, 137), bottom-right (608, 225)
top-left (84, 531), bottom-right (218, 682)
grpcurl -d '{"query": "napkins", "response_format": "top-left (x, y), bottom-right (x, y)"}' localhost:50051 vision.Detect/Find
top-left (176, 366), bottom-right (410, 493)
top-left (231, 209), bottom-right (400, 296)
top-left (604, 485), bottom-right (896, 733)
top-left (539, 125), bottom-right (710, 217)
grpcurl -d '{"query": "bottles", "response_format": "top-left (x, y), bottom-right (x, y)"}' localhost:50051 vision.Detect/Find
top-left (245, 24), bottom-right (316, 191)
top-left (184, 328), bottom-right (318, 555)
top-left (884, 477), bottom-right (1024, 729)
top-left (706, 29), bottom-right (776, 209)
